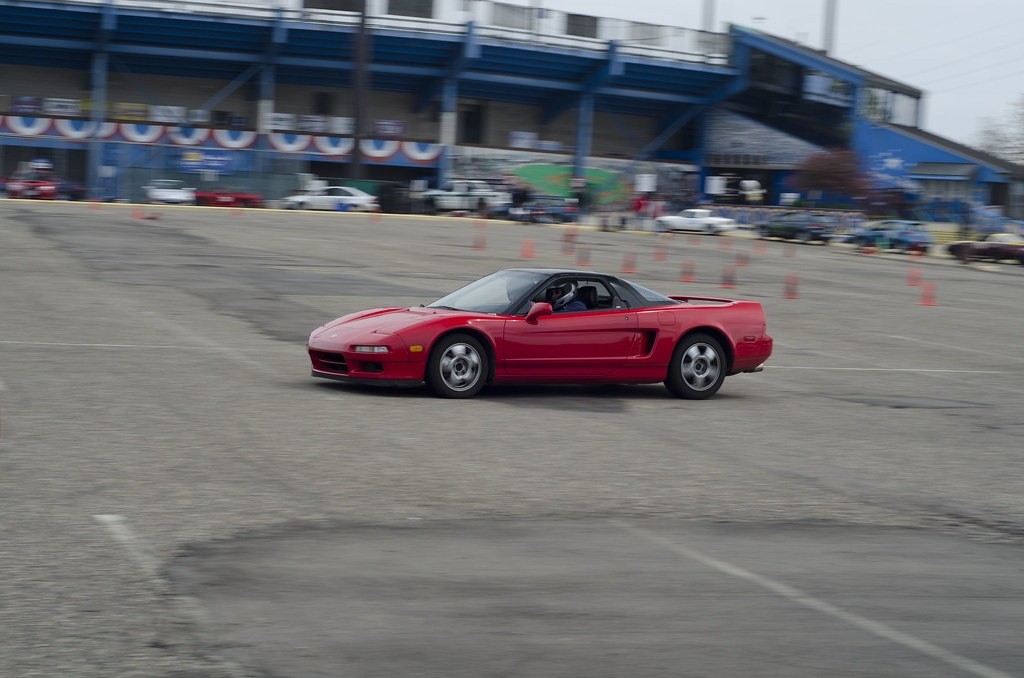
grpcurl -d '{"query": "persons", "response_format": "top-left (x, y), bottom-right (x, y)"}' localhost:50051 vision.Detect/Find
top-left (547, 280), bottom-right (587, 312)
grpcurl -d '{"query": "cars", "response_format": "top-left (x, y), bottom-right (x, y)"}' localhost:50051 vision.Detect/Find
top-left (306, 269), bottom-right (775, 400)
top-left (947, 233), bottom-right (1024, 262)
top-left (754, 211), bottom-right (837, 243)
top-left (847, 219), bottom-right (935, 255)
top-left (420, 180), bottom-right (582, 225)
top-left (141, 179), bottom-right (198, 206)
top-left (280, 186), bottom-right (380, 213)
top-left (1, 168), bottom-right (64, 201)
top-left (654, 209), bottom-right (737, 235)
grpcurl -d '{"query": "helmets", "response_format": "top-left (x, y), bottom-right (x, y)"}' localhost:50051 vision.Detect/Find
top-left (546, 279), bottom-right (578, 307)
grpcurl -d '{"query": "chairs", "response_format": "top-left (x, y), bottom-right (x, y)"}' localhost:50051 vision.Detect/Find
top-left (577, 286), bottom-right (598, 310)
